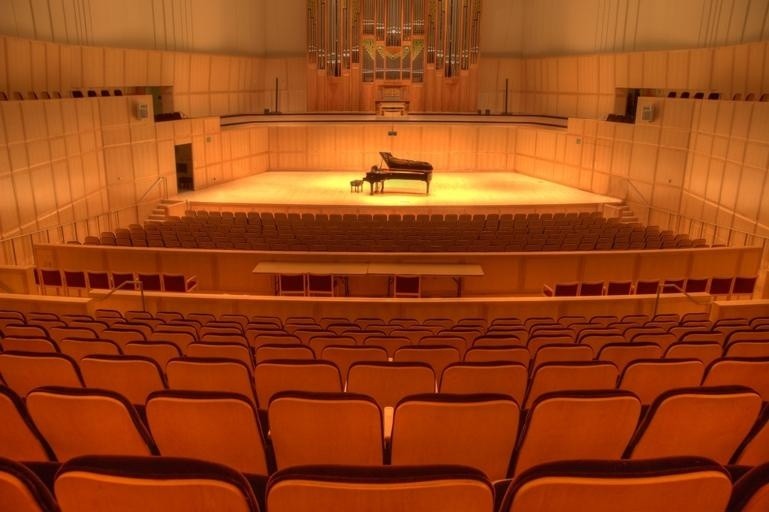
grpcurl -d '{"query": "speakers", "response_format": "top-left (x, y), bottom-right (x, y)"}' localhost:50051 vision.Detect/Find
top-left (642, 103), bottom-right (655, 122)
top-left (137, 104), bottom-right (148, 121)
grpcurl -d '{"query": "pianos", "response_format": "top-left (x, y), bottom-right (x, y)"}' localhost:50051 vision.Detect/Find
top-left (362, 152), bottom-right (433, 196)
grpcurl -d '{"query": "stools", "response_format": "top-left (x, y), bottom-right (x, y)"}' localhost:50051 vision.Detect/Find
top-left (350, 180), bottom-right (363, 192)
